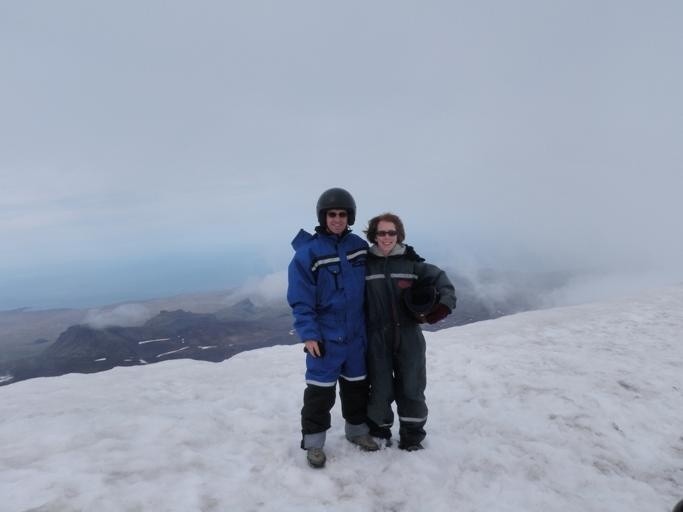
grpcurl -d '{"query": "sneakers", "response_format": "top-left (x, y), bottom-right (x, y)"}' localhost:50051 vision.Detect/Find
top-left (346, 433), bottom-right (379, 451)
top-left (308, 448), bottom-right (325, 466)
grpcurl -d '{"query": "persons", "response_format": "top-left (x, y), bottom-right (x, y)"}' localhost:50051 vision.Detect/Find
top-left (287, 188), bottom-right (379, 467)
top-left (363, 213), bottom-right (457, 450)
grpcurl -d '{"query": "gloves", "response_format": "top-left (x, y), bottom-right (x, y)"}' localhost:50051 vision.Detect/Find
top-left (427, 303), bottom-right (450, 324)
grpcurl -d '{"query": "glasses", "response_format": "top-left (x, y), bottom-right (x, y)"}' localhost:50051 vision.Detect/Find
top-left (327, 212), bottom-right (348, 217)
top-left (376, 230), bottom-right (397, 236)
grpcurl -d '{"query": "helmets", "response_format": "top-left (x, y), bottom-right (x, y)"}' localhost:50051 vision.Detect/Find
top-left (316, 188), bottom-right (356, 226)
top-left (403, 286), bottom-right (439, 323)
